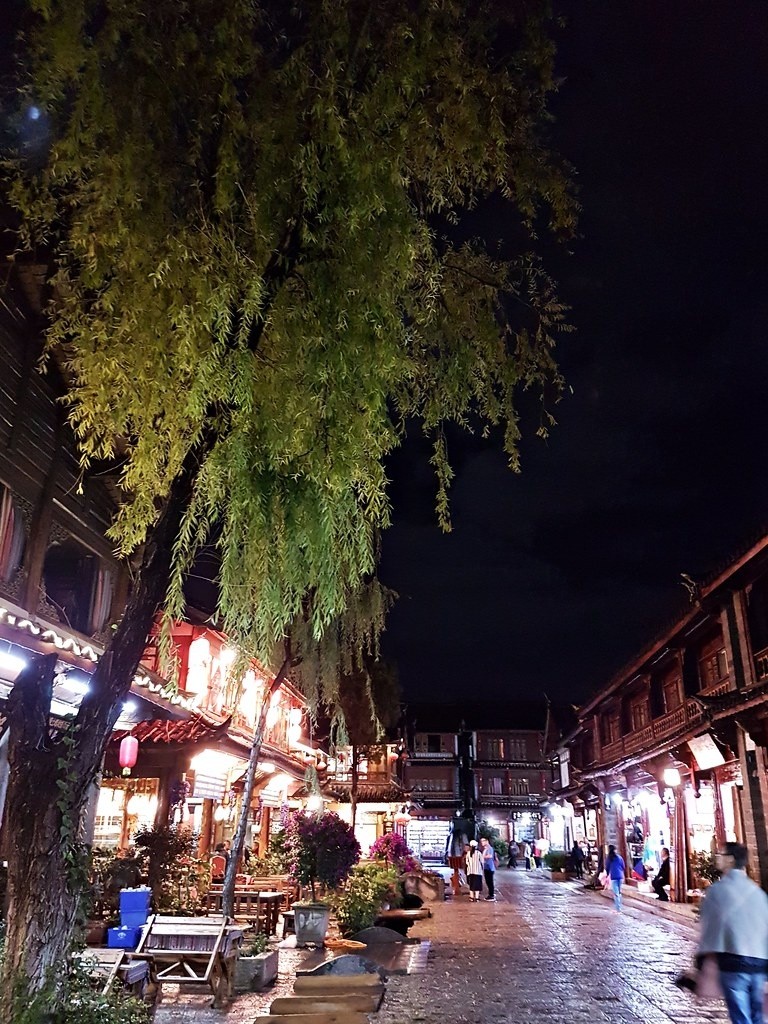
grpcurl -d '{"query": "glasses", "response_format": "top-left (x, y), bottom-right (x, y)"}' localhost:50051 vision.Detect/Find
top-left (715, 852), bottom-right (735, 858)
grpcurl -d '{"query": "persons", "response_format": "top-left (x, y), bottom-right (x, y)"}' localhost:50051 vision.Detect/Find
top-left (606, 844), bottom-right (626, 913)
top-left (691, 842), bottom-right (768, 1024)
top-left (461, 837), bottom-right (552, 902)
top-left (651, 848), bottom-right (672, 901)
top-left (570, 836), bottom-right (593, 882)
top-left (214, 839), bottom-right (260, 876)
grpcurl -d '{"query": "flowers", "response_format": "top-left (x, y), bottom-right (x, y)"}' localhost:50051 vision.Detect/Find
top-left (285, 808), bottom-right (360, 901)
top-left (370, 832), bottom-right (418, 874)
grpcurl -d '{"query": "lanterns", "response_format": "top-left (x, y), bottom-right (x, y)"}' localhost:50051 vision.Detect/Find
top-left (119, 735), bottom-right (138, 778)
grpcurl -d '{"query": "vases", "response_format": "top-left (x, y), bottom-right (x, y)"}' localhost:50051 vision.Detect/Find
top-left (292, 903), bottom-right (332, 949)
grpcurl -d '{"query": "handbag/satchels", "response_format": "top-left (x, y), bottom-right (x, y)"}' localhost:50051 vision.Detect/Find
top-left (605, 873), bottom-right (612, 891)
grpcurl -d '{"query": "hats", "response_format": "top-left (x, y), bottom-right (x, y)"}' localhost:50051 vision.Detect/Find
top-left (470, 840), bottom-right (478, 846)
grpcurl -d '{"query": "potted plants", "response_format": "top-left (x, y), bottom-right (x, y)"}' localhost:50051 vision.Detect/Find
top-left (335, 863), bottom-right (401, 935)
top-left (233, 933), bottom-right (279, 991)
top-left (545, 852), bottom-right (567, 882)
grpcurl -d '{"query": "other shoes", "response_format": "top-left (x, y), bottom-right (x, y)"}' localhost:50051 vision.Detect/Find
top-left (527, 869), bottom-right (531, 872)
top-left (476, 896), bottom-right (481, 902)
top-left (581, 877), bottom-right (586, 880)
top-left (469, 897), bottom-right (474, 902)
top-left (577, 876), bottom-right (580, 880)
top-left (484, 895), bottom-right (496, 902)
top-left (656, 895), bottom-right (669, 901)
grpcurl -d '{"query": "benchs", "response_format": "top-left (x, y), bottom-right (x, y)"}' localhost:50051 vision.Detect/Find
top-left (198, 886), bottom-right (297, 935)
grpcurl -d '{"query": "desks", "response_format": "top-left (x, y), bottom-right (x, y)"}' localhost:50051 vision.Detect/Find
top-left (206, 876), bottom-right (290, 932)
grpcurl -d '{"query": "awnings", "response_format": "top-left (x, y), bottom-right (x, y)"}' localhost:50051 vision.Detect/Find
top-left (0, 604), bottom-right (332, 797)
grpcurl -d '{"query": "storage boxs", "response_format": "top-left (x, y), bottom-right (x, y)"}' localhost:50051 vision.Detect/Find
top-left (107, 891), bottom-right (152, 948)
